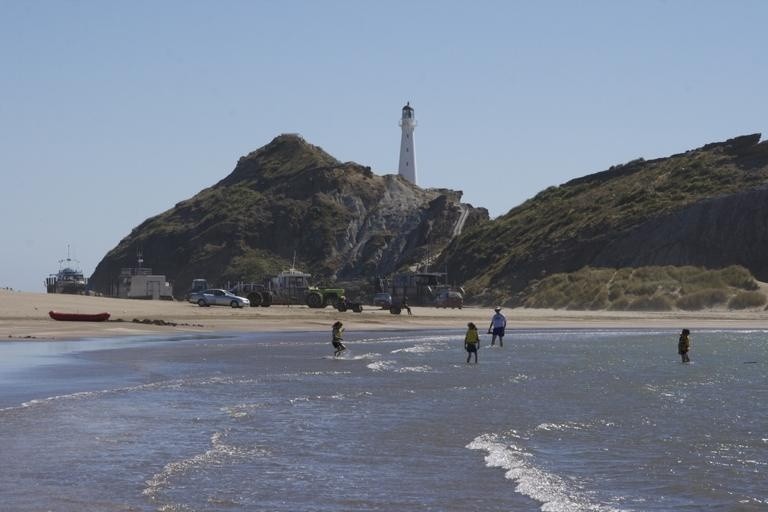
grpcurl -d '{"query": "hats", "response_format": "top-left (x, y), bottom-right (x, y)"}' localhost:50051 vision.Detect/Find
top-left (680, 328), bottom-right (690, 335)
top-left (494, 306), bottom-right (501, 311)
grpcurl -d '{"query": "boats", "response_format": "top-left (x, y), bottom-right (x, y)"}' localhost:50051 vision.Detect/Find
top-left (46, 310), bottom-right (112, 323)
top-left (43, 242), bottom-right (90, 294)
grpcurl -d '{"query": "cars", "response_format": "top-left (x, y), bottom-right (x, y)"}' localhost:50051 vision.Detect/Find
top-left (331, 297), bottom-right (352, 310)
top-left (372, 292), bottom-right (391, 306)
top-left (187, 287), bottom-right (251, 309)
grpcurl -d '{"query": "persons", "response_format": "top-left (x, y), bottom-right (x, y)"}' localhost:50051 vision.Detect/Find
top-left (384, 293), bottom-right (393, 306)
top-left (679, 329), bottom-right (691, 362)
top-left (404, 296), bottom-right (412, 315)
top-left (466, 322), bottom-right (478, 344)
top-left (333, 322), bottom-right (346, 357)
top-left (488, 305), bottom-right (506, 347)
top-left (464, 325), bottom-right (480, 363)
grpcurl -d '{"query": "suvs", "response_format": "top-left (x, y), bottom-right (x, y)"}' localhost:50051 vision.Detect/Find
top-left (433, 289), bottom-right (464, 310)
